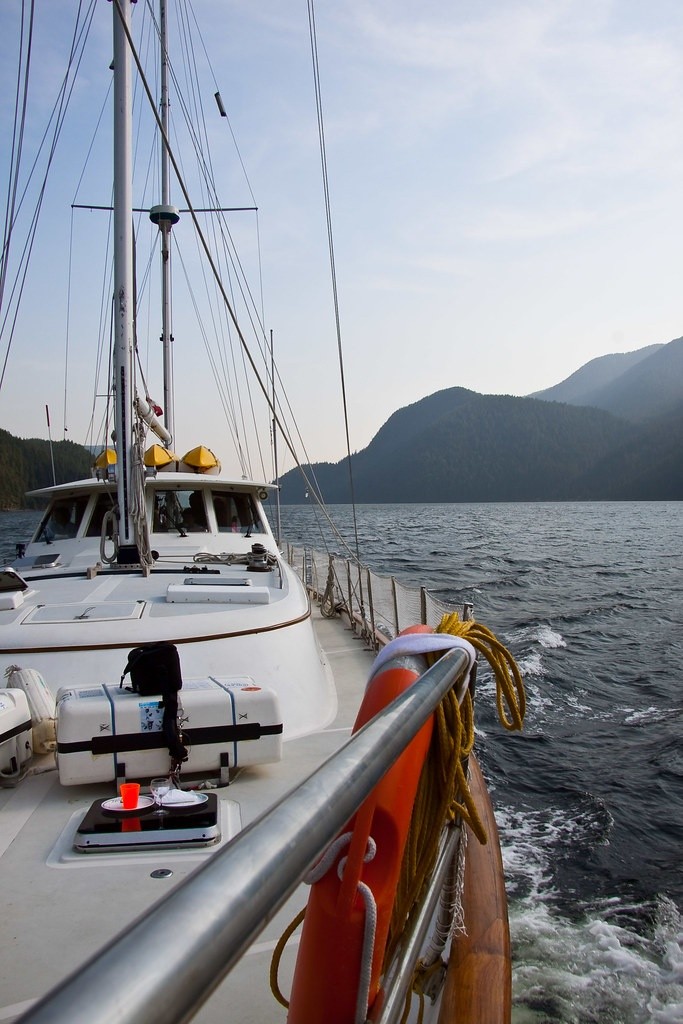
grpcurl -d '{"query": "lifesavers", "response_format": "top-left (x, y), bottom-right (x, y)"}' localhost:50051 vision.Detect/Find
top-left (285, 625), bottom-right (438, 1024)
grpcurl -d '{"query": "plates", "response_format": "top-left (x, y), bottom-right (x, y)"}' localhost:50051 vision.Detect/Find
top-left (156, 791), bottom-right (209, 807)
top-left (101, 795), bottom-right (154, 811)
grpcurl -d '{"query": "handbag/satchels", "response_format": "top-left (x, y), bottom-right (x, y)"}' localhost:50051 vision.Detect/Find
top-left (120, 641), bottom-right (189, 762)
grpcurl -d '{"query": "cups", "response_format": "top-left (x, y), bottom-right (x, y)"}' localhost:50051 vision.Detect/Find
top-left (120, 783), bottom-right (140, 809)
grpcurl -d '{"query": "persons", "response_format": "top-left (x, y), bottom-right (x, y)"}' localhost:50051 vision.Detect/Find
top-left (182, 493), bottom-right (240, 529)
top-left (42, 505), bottom-right (79, 539)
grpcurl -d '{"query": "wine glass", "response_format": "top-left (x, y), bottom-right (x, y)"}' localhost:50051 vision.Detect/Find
top-left (151, 778), bottom-right (170, 816)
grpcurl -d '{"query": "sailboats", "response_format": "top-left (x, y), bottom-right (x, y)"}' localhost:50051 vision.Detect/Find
top-left (0, 0), bottom-right (515, 1024)
